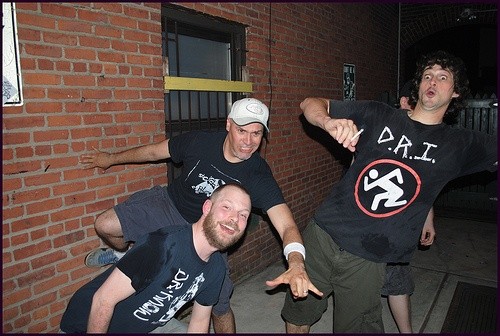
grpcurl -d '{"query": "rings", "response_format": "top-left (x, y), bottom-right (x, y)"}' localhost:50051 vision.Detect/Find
top-left (303, 291), bottom-right (308, 293)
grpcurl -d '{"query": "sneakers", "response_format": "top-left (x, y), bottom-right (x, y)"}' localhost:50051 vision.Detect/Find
top-left (84, 245), bottom-right (130, 268)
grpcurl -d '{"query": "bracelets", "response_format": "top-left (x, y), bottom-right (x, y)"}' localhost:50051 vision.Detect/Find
top-left (283, 242), bottom-right (306, 261)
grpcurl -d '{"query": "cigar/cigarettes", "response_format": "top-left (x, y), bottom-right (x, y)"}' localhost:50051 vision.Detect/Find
top-left (350, 128), bottom-right (364, 143)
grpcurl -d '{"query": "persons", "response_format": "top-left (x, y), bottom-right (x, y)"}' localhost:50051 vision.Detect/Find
top-left (350, 81), bottom-right (436, 334)
top-left (79, 98), bottom-right (323, 334)
top-left (58, 183), bottom-right (252, 334)
top-left (281, 50), bottom-right (500, 334)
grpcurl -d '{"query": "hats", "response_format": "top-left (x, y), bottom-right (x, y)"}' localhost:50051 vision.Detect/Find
top-left (229, 98), bottom-right (270, 132)
